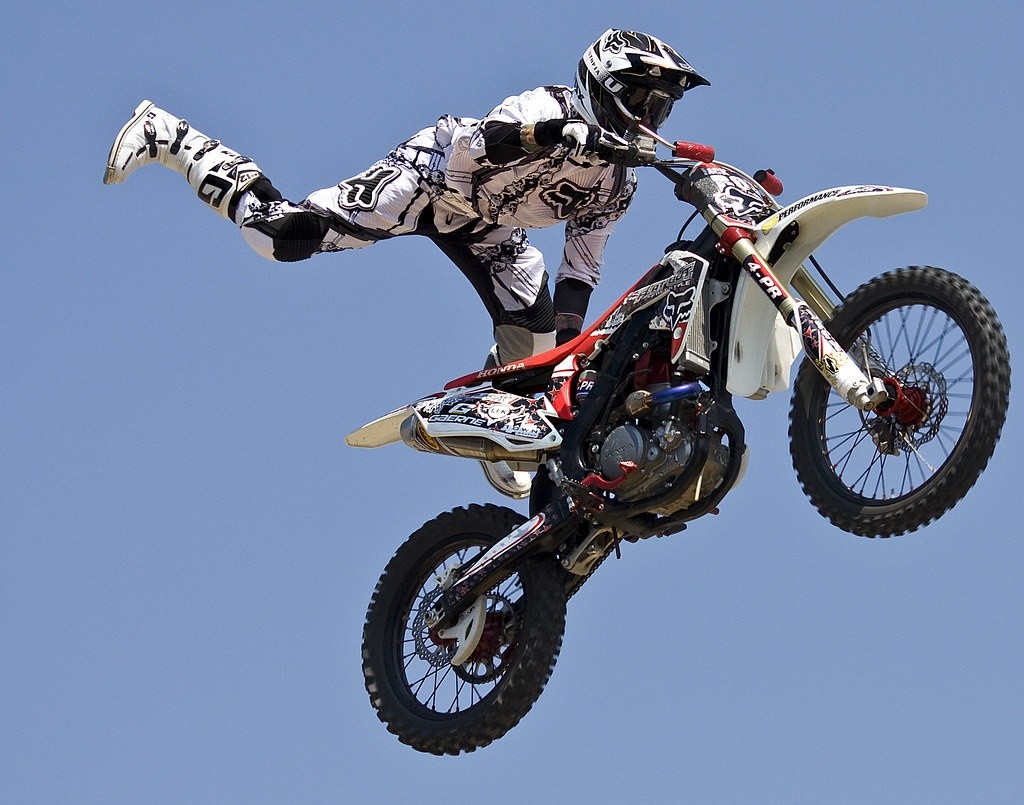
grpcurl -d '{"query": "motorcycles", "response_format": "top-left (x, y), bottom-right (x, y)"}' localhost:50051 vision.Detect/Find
top-left (335, 116), bottom-right (1011, 756)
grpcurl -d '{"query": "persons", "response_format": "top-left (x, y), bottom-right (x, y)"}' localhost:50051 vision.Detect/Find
top-left (102, 26), bottom-right (712, 499)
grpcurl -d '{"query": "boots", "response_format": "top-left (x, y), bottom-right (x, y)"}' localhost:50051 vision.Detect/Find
top-left (477, 342), bottom-right (532, 500)
top-left (102, 97), bottom-right (273, 225)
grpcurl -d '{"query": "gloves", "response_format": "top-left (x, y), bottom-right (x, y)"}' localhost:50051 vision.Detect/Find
top-left (561, 119), bottom-right (629, 158)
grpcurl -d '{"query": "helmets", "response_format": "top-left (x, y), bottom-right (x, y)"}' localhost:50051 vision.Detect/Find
top-left (569, 26), bottom-right (712, 153)
